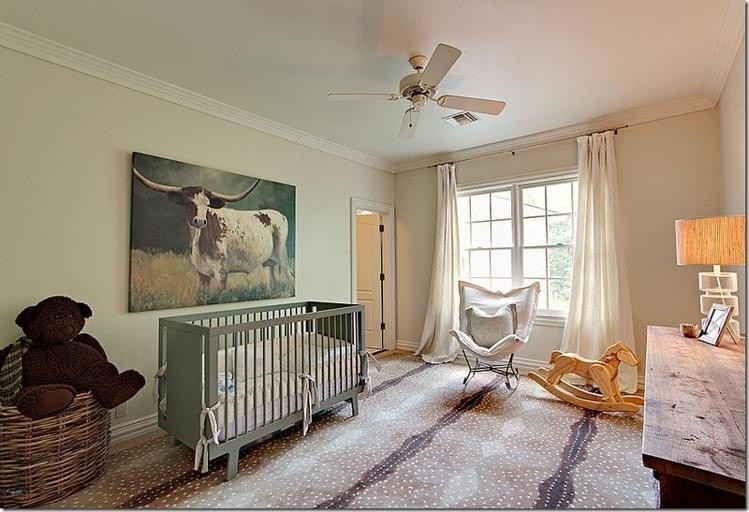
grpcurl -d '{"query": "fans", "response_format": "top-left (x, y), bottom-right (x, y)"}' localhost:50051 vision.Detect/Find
top-left (328, 44), bottom-right (505, 137)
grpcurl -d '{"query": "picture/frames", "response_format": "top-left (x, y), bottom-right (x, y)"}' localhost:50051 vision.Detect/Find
top-left (698, 303), bottom-right (737, 347)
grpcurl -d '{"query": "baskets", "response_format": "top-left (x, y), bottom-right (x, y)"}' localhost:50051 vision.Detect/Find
top-left (0, 390), bottom-right (111, 509)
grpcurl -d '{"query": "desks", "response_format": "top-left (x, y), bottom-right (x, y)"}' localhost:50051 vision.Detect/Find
top-left (642, 326), bottom-right (747, 508)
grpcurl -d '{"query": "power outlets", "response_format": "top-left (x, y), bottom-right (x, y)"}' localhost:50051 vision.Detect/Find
top-left (116, 404), bottom-right (128, 417)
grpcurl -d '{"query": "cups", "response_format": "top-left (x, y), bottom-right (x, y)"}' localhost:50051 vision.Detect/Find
top-left (681, 323), bottom-right (698, 337)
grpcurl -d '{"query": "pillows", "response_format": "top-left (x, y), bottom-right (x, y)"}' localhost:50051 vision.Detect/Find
top-left (465, 304), bottom-right (517, 348)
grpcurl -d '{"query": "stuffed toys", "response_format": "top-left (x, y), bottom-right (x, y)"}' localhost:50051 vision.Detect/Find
top-left (11, 295), bottom-right (146, 420)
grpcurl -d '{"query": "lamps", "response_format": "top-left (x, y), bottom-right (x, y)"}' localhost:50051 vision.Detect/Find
top-left (675, 214), bottom-right (745, 342)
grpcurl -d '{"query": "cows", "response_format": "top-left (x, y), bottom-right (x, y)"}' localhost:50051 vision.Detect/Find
top-left (130, 165), bottom-right (294, 305)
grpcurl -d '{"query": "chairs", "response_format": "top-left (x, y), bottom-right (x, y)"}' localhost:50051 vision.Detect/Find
top-left (448, 280), bottom-right (540, 391)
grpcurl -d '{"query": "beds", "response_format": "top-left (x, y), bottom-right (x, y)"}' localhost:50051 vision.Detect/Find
top-left (155, 301), bottom-right (381, 481)
